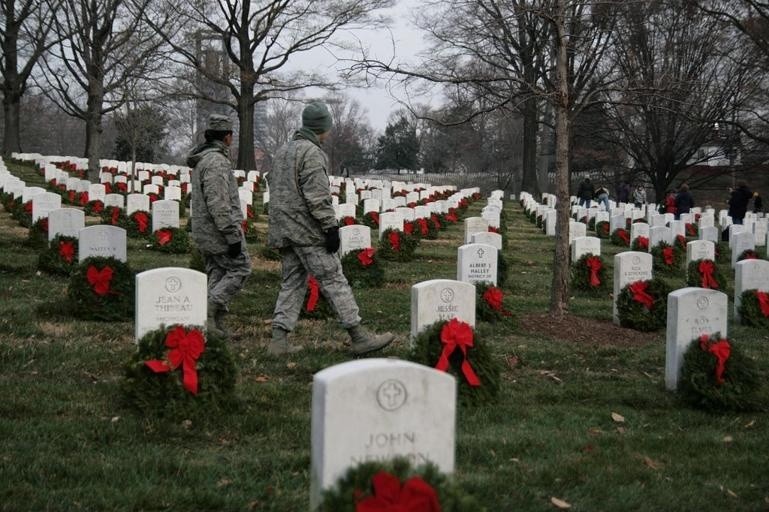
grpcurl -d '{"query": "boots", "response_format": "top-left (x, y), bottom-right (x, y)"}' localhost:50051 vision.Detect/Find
top-left (348, 323), bottom-right (394, 355)
top-left (207, 301), bottom-right (231, 339)
top-left (266, 325), bottom-right (304, 360)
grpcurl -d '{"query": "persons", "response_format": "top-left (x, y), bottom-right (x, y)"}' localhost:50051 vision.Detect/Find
top-left (576, 173), bottom-right (597, 208)
top-left (265, 99), bottom-right (397, 360)
top-left (663, 186), bottom-right (678, 216)
top-left (753, 192), bottom-right (762, 213)
top-left (632, 185), bottom-right (647, 209)
top-left (185, 112), bottom-right (254, 340)
top-left (594, 186), bottom-right (610, 211)
top-left (675, 182), bottom-right (696, 221)
top-left (727, 180), bottom-right (753, 224)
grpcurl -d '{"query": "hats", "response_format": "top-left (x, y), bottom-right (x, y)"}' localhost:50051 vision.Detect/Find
top-left (302, 102), bottom-right (333, 135)
top-left (207, 113), bottom-right (233, 131)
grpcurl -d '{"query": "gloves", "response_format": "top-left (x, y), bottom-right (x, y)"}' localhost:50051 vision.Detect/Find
top-left (325, 226), bottom-right (341, 255)
top-left (228, 242), bottom-right (242, 260)
